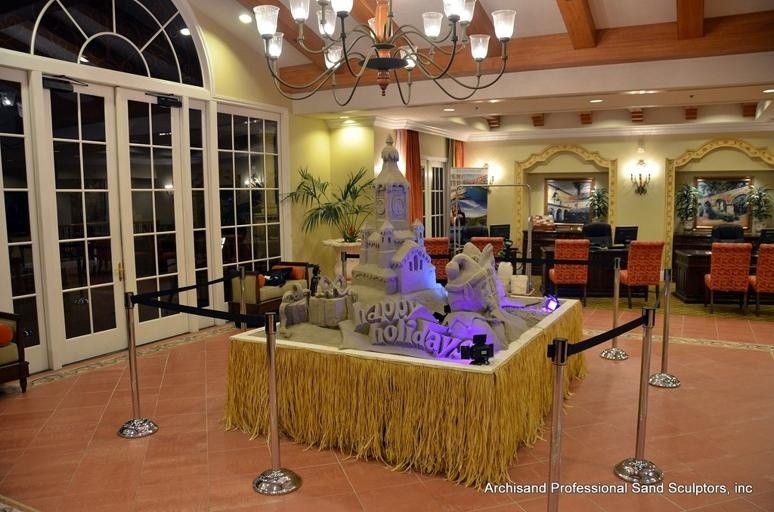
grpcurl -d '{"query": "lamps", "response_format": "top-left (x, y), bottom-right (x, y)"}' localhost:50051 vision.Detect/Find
top-left (252, 1), bottom-right (515, 105)
top-left (630, 160), bottom-right (651, 195)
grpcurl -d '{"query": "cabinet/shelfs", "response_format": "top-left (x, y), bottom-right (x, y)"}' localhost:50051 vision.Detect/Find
top-left (522, 231), bottom-right (586, 275)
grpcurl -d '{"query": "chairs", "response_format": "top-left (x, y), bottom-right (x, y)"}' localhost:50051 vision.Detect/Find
top-left (423, 236), bottom-right (774, 317)
top-left (583, 222), bottom-right (613, 248)
top-left (713, 224), bottom-right (745, 238)
top-left (460, 225), bottom-right (489, 248)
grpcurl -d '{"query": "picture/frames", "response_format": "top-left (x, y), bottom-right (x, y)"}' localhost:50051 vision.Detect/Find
top-left (544, 177), bottom-right (595, 227)
top-left (692, 176), bottom-right (753, 233)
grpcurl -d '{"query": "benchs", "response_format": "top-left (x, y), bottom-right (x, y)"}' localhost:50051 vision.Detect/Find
top-left (229, 261), bottom-right (321, 328)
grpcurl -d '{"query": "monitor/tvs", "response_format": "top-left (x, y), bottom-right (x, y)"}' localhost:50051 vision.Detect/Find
top-left (490, 224), bottom-right (510, 239)
top-left (615, 226), bottom-right (638, 248)
top-left (759, 229), bottom-right (774, 244)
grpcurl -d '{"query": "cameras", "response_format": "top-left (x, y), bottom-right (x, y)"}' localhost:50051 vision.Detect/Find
top-left (461, 335), bottom-right (494, 364)
